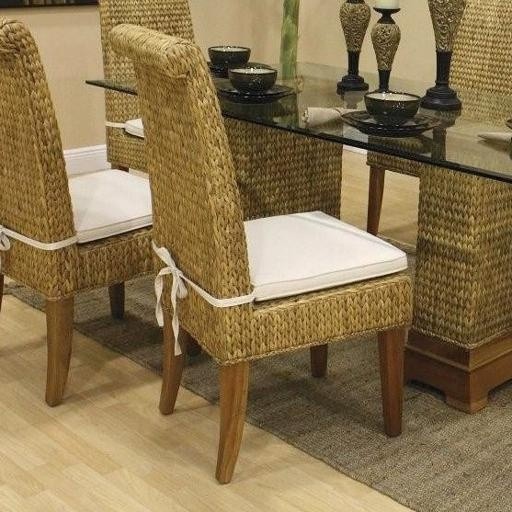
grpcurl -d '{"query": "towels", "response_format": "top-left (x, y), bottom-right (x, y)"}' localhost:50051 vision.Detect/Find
top-left (302, 107), bottom-right (362, 130)
top-left (476, 132), bottom-right (512, 147)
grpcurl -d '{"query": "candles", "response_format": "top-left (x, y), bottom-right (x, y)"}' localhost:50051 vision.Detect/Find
top-left (374, 0), bottom-right (399, 9)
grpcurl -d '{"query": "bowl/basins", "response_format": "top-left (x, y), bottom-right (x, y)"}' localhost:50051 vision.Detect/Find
top-left (365, 91), bottom-right (421, 126)
top-left (227, 67), bottom-right (276, 94)
top-left (208, 46), bottom-right (249, 66)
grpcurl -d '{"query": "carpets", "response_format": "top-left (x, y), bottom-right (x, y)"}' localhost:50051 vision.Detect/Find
top-left (0, 231), bottom-right (511, 511)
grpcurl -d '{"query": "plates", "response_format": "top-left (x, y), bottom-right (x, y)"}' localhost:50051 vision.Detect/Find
top-left (215, 85), bottom-right (293, 101)
top-left (343, 110), bottom-right (441, 136)
top-left (207, 61), bottom-right (271, 77)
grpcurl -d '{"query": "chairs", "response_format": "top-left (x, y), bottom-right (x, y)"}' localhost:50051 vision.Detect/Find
top-left (0, 17), bottom-right (151, 406)
top-left (109, 25), bottom-right (414, 484)
top-left (367, 0), bottom-right (511, 235)
top-left (98, 0), bottom-right (195, 318)
